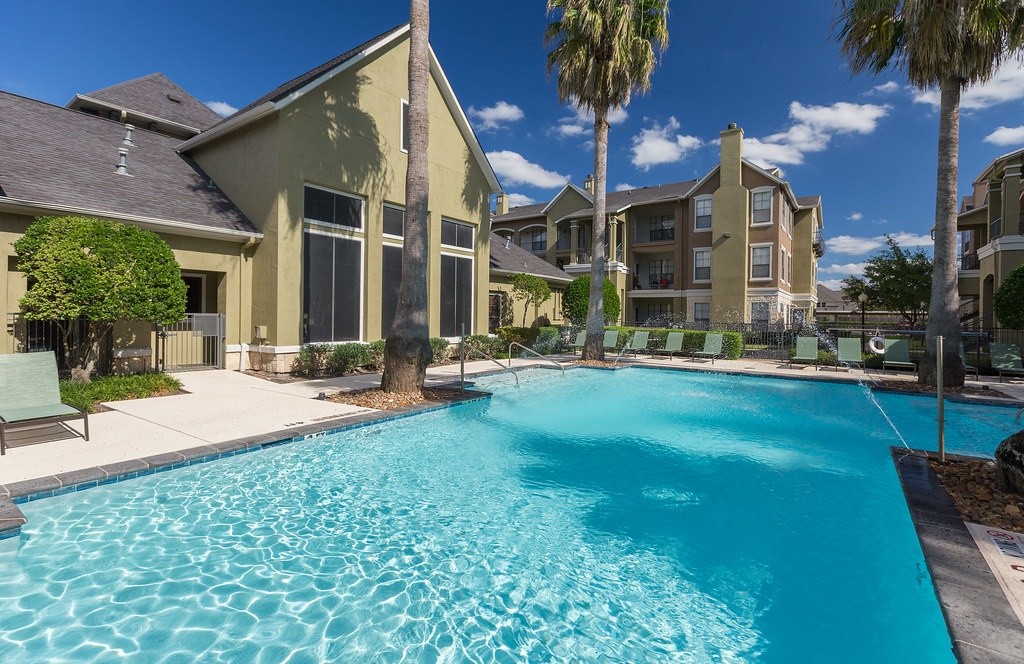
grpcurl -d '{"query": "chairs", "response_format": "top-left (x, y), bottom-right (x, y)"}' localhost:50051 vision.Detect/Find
top-left (0, 350), bottom-right (90, 456)
top-left (652, 332), bottom-right (685, 361)
top-left (835, 337), bottom-right (865, 373)
top-left (692, 333), bottom-right (725, 364)
top-left (882, 339), bottom-right (917, 378)
top-left (989, 342), bottom-right (1023, 384)
top-left (650, 279), bottom-right (668, 289)
top-left (789, 336), bottom-right (819, 372)
top-left (959, 341), bottom-right (979, 380)
top-left (626, 332), bottom-right (651, 358)
top-left (567, 330), bottom-right (620, 356)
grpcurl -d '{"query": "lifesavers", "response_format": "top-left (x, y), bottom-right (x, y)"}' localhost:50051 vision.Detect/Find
top-left (869, 336), bottom-right (888, 355)
